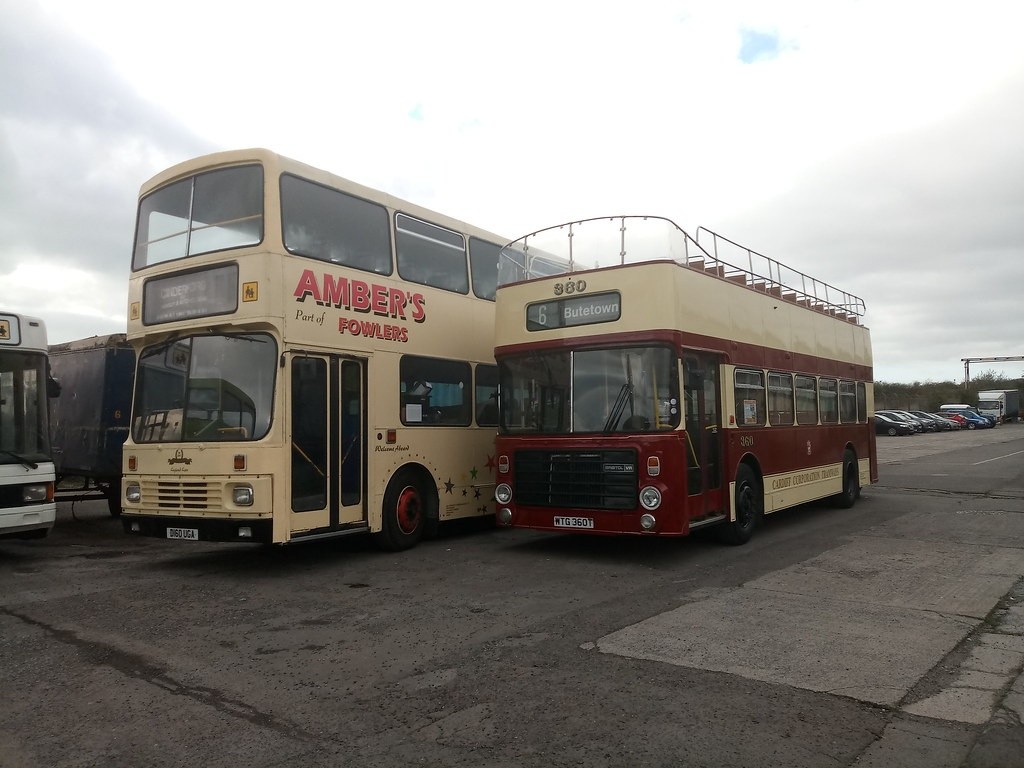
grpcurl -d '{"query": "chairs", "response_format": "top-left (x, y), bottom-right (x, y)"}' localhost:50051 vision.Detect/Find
top-left (683, 258), bottom-right (860, 325)
top-left (736, 404), bottom-right (869, 425)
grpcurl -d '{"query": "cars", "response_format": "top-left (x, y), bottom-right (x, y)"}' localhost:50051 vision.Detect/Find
top-left (892, 409), bottom-right (935, 433)
top-left (937, 413), bottom-right (966, 428)
top-left (910, 410), bottom-right (947, 433)
top-left (960, 414), bottom-right (979, 430)
top-left (875, 411), bottom-right (923, 434)
top-left (875, 415), bottom-right (914, 437)
top-left (948, 410), bottom-right (989, 429)
top-left (928, 413), bottom-right (962, 432)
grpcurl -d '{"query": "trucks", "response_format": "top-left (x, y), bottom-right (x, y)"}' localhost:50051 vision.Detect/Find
top-left (977, 390), bottom-right (1020, 425)
top-left (940, 404), bottom-right (999, 430)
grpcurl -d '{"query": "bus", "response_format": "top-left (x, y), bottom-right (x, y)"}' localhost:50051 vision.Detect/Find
top-left (493, 215), bottom-right (881, 542)
top-left (113, 145), bottom-right (600, 550)
top-left (0, 310), bottom-right (62, 544)
